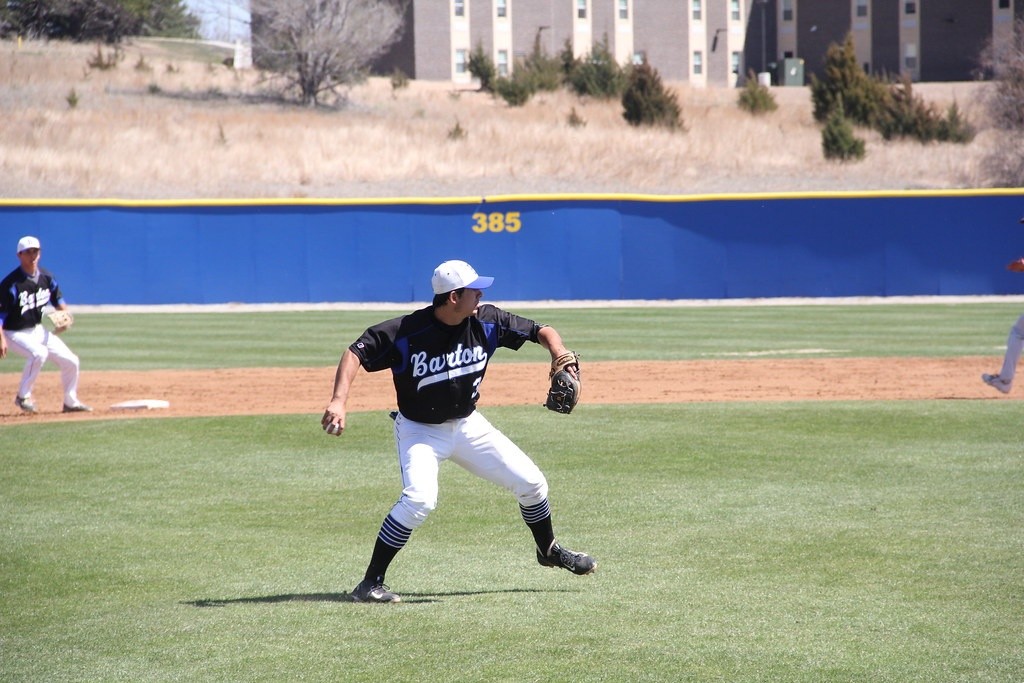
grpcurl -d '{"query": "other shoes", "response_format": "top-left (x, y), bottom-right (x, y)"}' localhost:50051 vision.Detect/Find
top-left (15, 395), bottom-right (41, 415)
top-left (62, 404), bottom-right (93, 412)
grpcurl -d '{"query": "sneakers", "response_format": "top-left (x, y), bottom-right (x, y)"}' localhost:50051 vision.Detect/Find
top-left (350, 576), bottom-right (401, 605)
top-left (536, 542), bottom-right (598, 575)
top-left (983, 372), bottom-right (1012, 394)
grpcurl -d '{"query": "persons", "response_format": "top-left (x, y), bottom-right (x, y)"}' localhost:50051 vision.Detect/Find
top-left (0, 236), bottom-right (93, 414)
top-left (981, 257), bottom-right (1024, 394)
top-left (321, 260), bottom-right (598, 603)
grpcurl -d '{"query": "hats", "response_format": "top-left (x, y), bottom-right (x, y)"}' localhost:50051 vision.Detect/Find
top-left (16, 235), bottom-right (42, 252)
top-left (431, 259), bottom-right (495, 295)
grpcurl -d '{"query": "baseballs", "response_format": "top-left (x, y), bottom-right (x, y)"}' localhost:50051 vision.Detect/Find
top-left (326, 422), bottom-right (339, 434)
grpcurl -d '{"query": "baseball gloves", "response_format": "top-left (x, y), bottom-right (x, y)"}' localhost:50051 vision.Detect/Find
top-left (47, 310), bottom-right (74, 335)
top-left (543, 350), bottom-right (582, 415)
